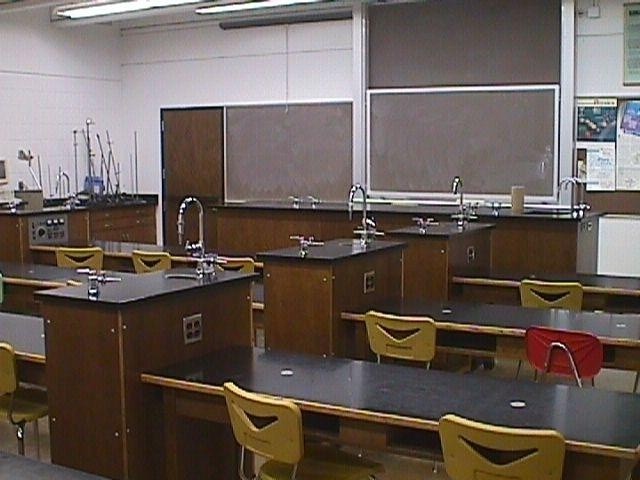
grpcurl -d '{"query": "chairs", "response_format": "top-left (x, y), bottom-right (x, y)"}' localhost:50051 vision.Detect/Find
top-left (437, 411), bottom-right (565, 479)
top-left (364, 310), bottom-right (437, 370)
top-left (220, 380), bottom-right (386, 479)
top-left (132, 247), bottom-right (172, 274)
top-left (0, 340), bottom-right (49, 457)
top-left (54, 245), bottom-right (104, 270)
top-left (519, 278), bottom-right (584, 312)
top-left (525, 325), bottom-right (604, 388)
top-left (218, 256), bottom-right (255, 347)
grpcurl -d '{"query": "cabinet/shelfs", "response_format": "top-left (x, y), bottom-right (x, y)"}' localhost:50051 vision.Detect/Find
top-left (88, 205), bottom-right (155, 246)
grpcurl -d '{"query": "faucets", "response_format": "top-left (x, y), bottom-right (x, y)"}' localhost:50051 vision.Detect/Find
top-left (451, 175), bottom-right (464, 215)
top-left (557, 179), bottom-right (576, 209)
top-left (176, 193), bottom-right (206, 254)
top-left (347, 183), bottom-right (376, 234)
top-left (566, 177), bottom-right (584, 206)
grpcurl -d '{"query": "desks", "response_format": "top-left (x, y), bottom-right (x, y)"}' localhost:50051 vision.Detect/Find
top-left (141, 343), bottom-right (640, 480)
top-left (340, 295), bottom-right (639, 370)
top-left (1, 262), bottom-right (261, 479)
top-left (452, 267), bottom-right (639, 312)
